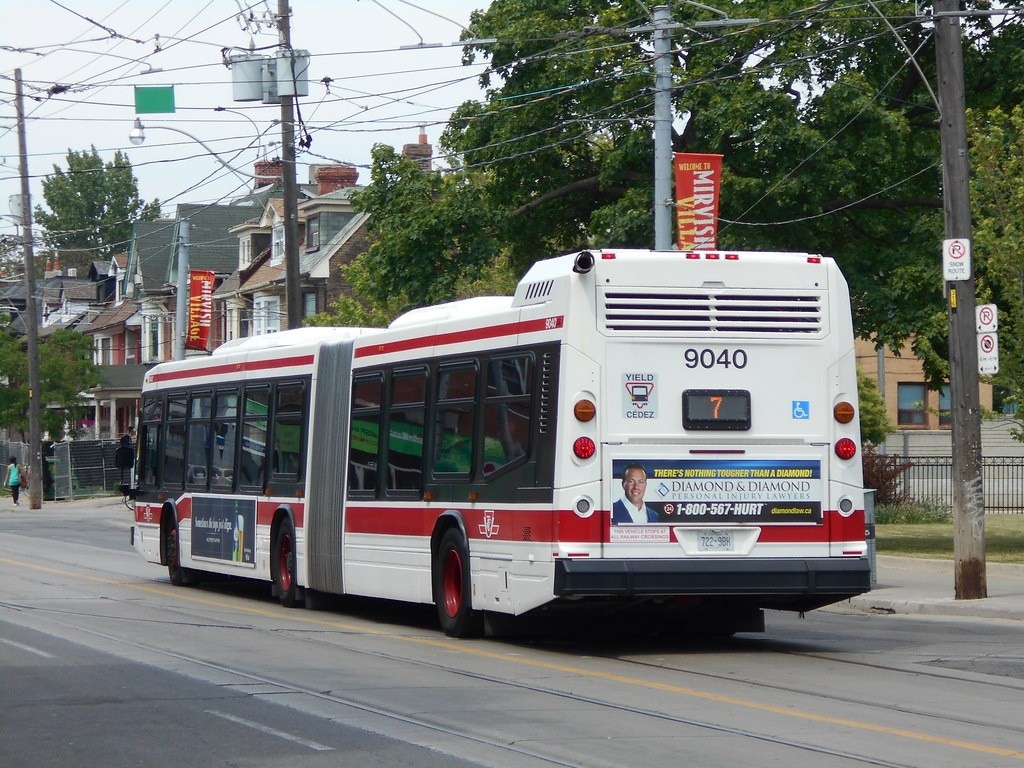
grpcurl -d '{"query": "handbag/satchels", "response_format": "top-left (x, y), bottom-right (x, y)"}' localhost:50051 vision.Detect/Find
top-left (20, 475), bottom-right (27, 488)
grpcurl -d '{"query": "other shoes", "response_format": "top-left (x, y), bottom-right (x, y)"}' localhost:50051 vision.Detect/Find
top-left (14, 502), bottom-right (19, 505)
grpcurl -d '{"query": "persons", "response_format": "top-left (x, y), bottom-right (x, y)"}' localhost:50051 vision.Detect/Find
top-left (4, 456), bottom-right (28, 506)
top-left (613, 463), bottom-right (659, 524)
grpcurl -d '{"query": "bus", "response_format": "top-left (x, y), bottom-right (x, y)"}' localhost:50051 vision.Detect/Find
top-left (116, 248), bottom-right (871, 638)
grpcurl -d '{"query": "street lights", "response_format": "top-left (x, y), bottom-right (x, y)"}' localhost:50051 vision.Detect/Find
top-left (129, 117), bottom-right (305, 331)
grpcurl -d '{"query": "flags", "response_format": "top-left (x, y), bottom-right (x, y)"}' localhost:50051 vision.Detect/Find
top-left (185, 269), bottom-right (216, 352)
top-left (674, 150), bottom-right (725, 248)
